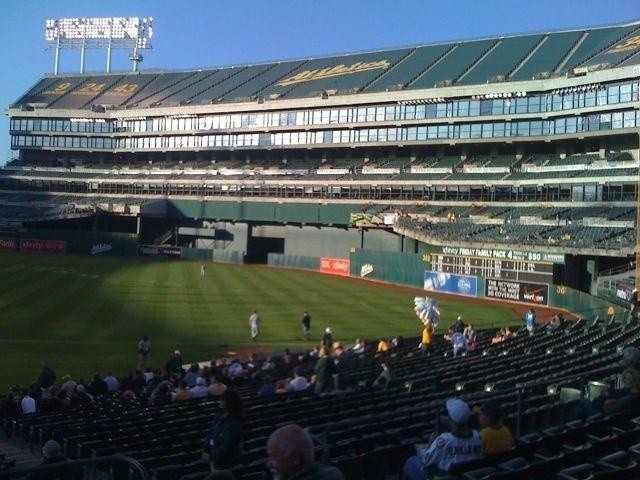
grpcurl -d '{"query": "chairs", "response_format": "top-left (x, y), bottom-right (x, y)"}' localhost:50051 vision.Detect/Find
top-left (13, 25), bottom-right (640, 109)
top-left (0, 154), bottom-right (640, 480)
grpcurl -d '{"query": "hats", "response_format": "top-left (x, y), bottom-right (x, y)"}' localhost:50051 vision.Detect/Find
top-left (445, 397), bottom-right (470, 424)
top-left (473, 400), bottom-right (502, 421)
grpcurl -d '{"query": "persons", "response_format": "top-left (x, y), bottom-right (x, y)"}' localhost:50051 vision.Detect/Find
top-left (0, 310), bottom-right (403, 415)
top-left (603, 346), bottom-right (640, 394)
top-left (206, 471), bottom-right (238, 480)
top-left (452, 213), bottom-right (455, 223)
top-left (499, 227), bottom-right (512, 241)
top-left (38, 439), bottom-right (81, 480)
top-left (592, 367), bottom-right (640, 415)
top-left (393, 208), bottom-right (423, 232)
top-left (265, 423), bottom-right (346, 480)
top-left (200, 264), bottom-right (208, 278)
top-left (418, 309), bottom-right (565, 358)
top-left (473, 399), bottom-right (517, 455)
top-left (426, 214), bottom-right (433, 230)
top-left (403, 397), bottom-right (483, 480)
top-left (548, 231), bottom-right (575, 246)
top-left (201, 388), bottom-right (247, 475)
top-left (447, 211), bottom-right (451, 222)
